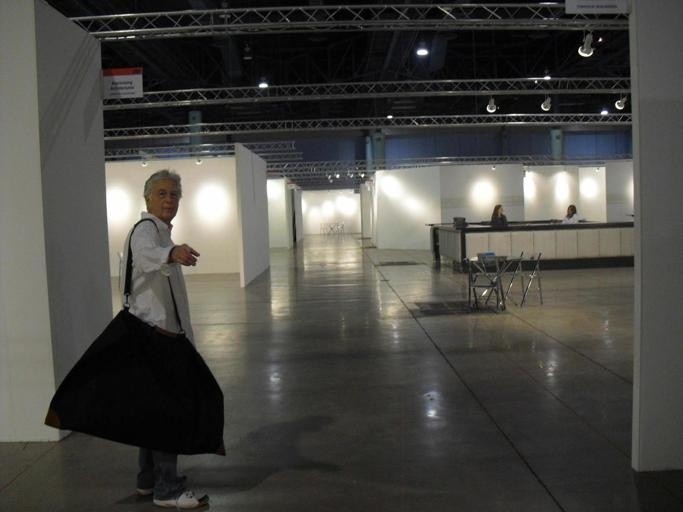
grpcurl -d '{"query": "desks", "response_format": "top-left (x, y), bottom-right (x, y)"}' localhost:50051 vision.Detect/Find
top-left (469, 255), bottom-right (521, 312)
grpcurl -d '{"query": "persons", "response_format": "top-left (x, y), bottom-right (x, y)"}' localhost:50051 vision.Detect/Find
top-left (562, 204), bottom-right (578, 223)
top-left (119, 169), bottom-right (211, 507)
top-left (489, 204), bottom-right (507, 225)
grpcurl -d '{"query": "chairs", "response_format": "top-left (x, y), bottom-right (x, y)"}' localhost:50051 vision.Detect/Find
top-left (478, 253), bottom-right (520, 307)
top-left (463, 257), bottom-right (499, 311)
top-left (503, 251), bottom-right (543, 308)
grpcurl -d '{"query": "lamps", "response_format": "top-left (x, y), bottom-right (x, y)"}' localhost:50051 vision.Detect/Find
top-left (541, 90), bottom-right (551, 111)
top-left (577, 24), bottom-right (594, 58)
top-left (486, 95), bottom-right (497, 113)
top-left (614, 94), bottom-right (628, 110)
top-left (416, 38), bottom-right (429, 55)
top-left (258, 73), bottom-right (268, 89)
top-left (386, 109), bottom-right (393, 119)
top-left (325, 170), bottom-right (365, 179)
top-left (242, 45), bottom-right (253, 60)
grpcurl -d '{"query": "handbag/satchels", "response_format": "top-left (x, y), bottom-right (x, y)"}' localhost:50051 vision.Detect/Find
top-left (43, 309), bottom-right (228, 457)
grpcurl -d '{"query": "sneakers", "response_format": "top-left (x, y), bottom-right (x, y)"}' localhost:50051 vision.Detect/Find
top-left (135, 471), bottom-right (210, 508)
